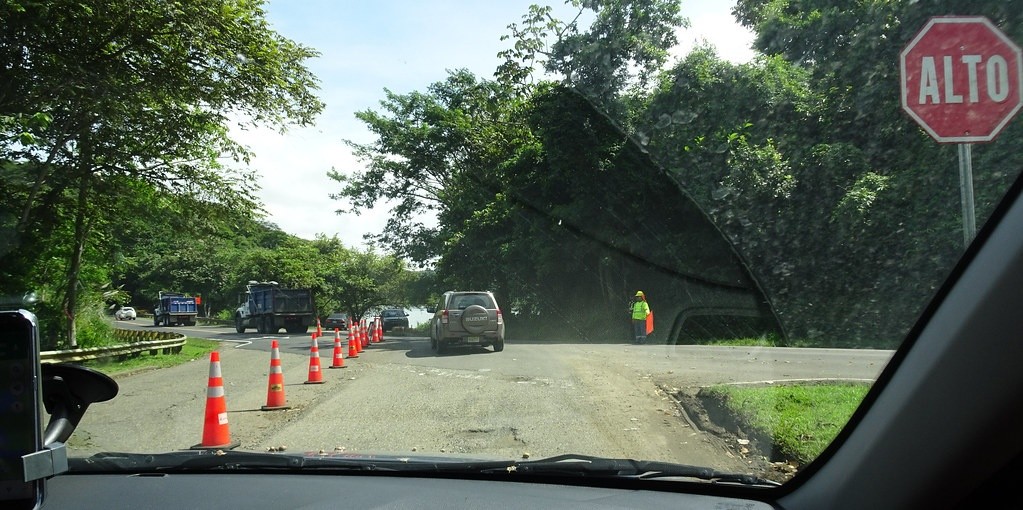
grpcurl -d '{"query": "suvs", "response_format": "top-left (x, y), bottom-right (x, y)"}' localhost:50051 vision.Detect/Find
top-left (427, 288), bottom-right (506, 355)
top-left (115, 307), bottom-right (136, 320)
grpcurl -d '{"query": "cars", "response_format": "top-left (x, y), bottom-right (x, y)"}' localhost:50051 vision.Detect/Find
top-left (325, 314), bottom-right (349, 330)
top-left (315, 316), bottom-right (323, 337)
top-left (380, 309), bottom-right (410, 332)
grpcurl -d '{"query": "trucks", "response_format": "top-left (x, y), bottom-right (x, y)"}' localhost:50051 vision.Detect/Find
top-left (235, 280), bottom-right (315, 333)
top-left (153, 290), bottom-right (198, 326)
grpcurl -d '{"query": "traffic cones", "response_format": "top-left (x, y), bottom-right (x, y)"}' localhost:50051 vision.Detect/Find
top-left (345, 318), bottom-right (360, 358)
top-left (303, 333), bottom-right (326, 384)
top-left (328, 328), bottom-right (348, 367)
top-left (371, 318), bottom-right (380, 343)
top-left (354, 321), bottom-right (365, 353)
top-left (260, 340), bottom-right (291, 410)
top-left (364, 319), bottom-right (371, 346)
top-left (361, 320), bottom-right (368, 348)
top-left (190, 352), bottom-right (241, 450)
top-left (379, 319), bottom-right (385, 342)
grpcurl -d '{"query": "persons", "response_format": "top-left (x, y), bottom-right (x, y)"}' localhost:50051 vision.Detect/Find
top-left (627, 291), bottom-right (650, 345)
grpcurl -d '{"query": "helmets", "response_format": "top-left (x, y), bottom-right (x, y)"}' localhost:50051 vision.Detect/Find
top-left (635, 291), bottom-right (644, 297)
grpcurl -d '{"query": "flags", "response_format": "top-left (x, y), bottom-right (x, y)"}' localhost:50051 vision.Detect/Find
top-left (645, 313), bottom-right (654, 335)
